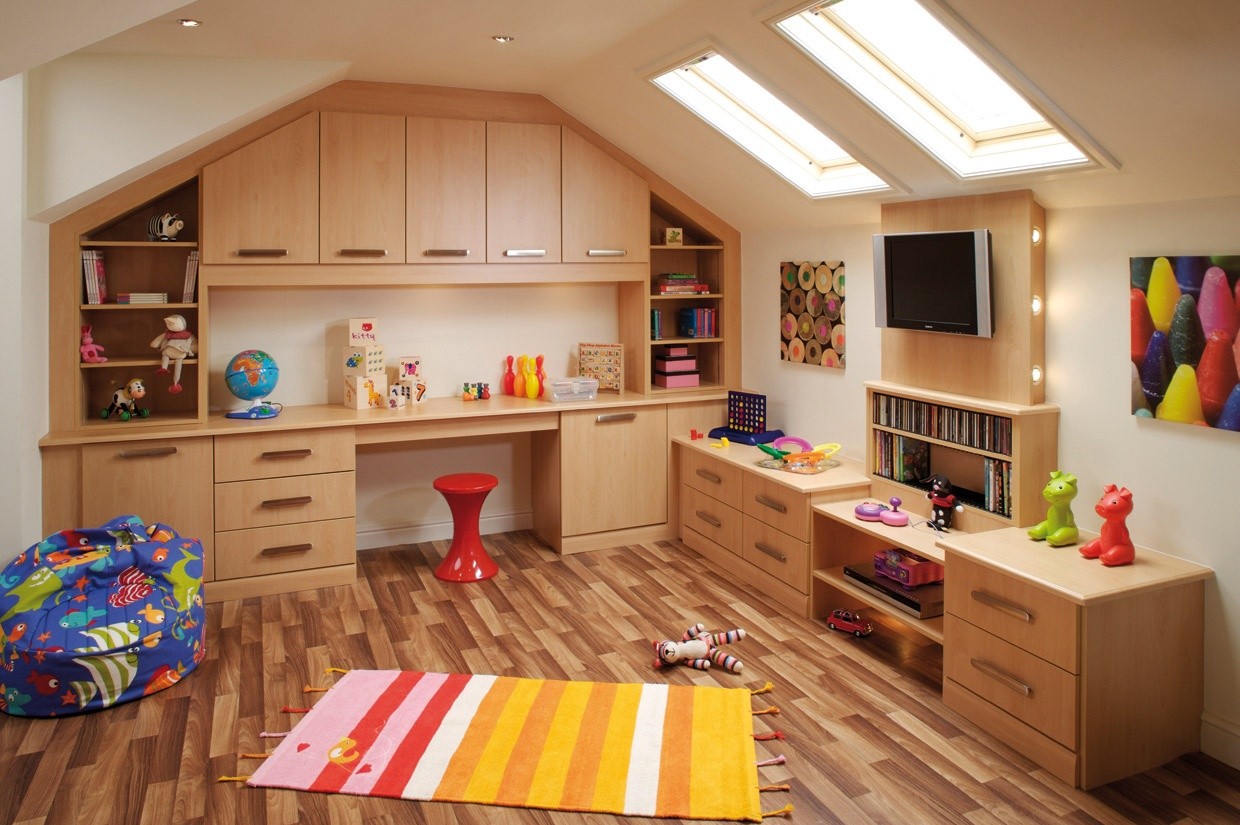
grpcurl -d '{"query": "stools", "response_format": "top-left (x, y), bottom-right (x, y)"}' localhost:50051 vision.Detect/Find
top-left (433, 472), bottom-right (499, 581)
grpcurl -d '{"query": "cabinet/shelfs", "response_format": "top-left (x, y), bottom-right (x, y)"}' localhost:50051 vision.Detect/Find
top-left (812, 495), bottom-right (973, 648)
top-left (404, 113), bottom-right (487, 286)
top-left (205, 105), bottom-right (320, 288)
top-left (85, 172), bottom-right (207, 425)
top-left (862, 380), bottom-right (1060, 536)
top-left (483, 119), bottom-right (561, 285)
top-left (317, 108), bottom-right (405, 287)
top-left (80, 442), bottom-right (215, 604)
top-left (626, 187), bottom-right (724, 394)
top-left (558, 125), bottom-right (651, 286)
top-left (532, 412), bottom-right (670, 555)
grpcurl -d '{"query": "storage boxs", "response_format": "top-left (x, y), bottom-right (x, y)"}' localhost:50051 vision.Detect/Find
top-left (664, 344), bottom-right (686, 355)
top-left (656, 354), bottom-right (697, 371)
top-left (654, 369), bottom-right (700, 388)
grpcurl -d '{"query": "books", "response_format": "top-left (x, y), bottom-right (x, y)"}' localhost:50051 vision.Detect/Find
top-left (870, 391), bottom-right (1013, 519)
top-left (651, 271), bottom-right (719, 342)
top-left (83, 248), bottom-right (200, 304)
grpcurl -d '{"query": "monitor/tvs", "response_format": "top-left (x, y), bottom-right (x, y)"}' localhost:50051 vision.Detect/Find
top-left (872, 229), bottom-right (993, 339)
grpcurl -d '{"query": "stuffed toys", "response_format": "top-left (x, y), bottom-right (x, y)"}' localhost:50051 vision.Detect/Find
top-left (652, 622), bottom-right (747, 676)
top-left (79, 324), bottom-right (108, 362)
top-left (919, 472), bottom-right (963, 532)
top-left (149, 314), bottom-right (198, 393)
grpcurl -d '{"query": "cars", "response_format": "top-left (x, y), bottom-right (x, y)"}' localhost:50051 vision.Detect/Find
top-left (828, 608), bottom-right (874, 641)
